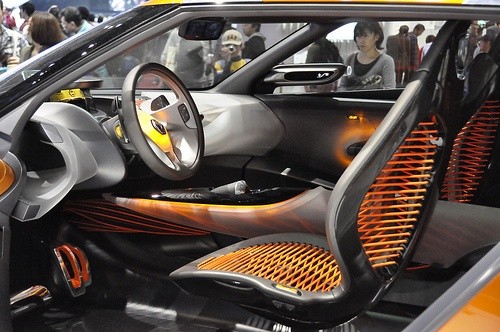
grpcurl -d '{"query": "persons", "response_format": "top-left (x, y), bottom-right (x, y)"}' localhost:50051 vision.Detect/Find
top-left (338, 22), bottom-right (396, 90)
top-left (0, 0), bottom-right (267, 88)
top-left (306, 35), bottom-right (344, 63)
top-left (386, 22), bottom-right (500, 85)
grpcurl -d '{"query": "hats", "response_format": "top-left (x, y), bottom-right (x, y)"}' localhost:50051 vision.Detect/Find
top-left (221, 29), bottom-right (244, 46)
top-left (477, 33), bottom-right (496, 42)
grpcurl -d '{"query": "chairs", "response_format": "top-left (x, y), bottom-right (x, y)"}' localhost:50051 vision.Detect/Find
top-left (438, 70), bottom-right (500, 205)
top-left (167, 111), bottom-right (452, 325)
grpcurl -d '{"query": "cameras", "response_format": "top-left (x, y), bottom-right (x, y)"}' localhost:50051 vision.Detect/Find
top-left (227, 45), bottom-right (236, 53)
